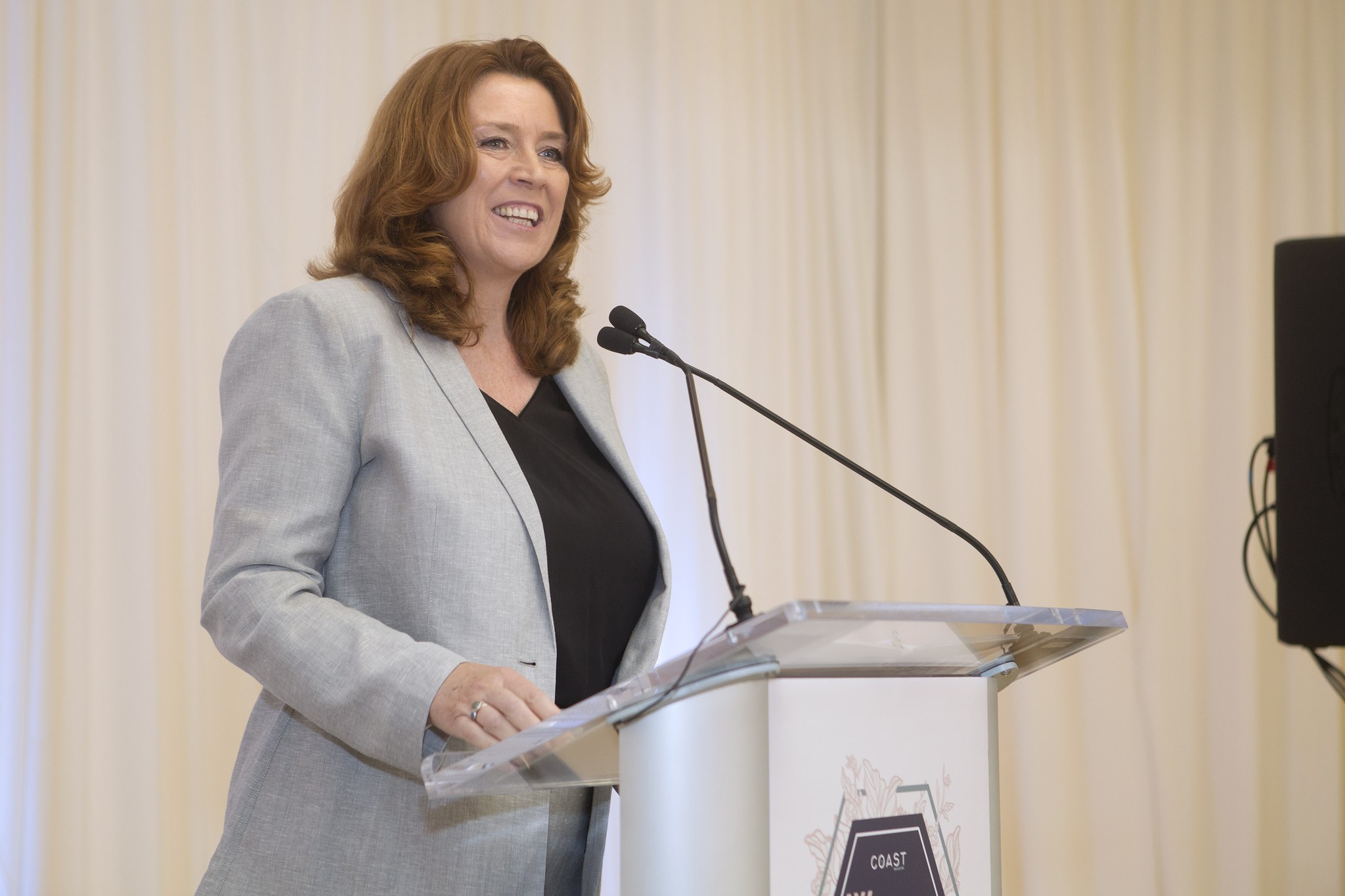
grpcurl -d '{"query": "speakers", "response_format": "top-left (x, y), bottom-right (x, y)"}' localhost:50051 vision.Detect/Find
top-left (1272, 233), bottom-right (1345, 648)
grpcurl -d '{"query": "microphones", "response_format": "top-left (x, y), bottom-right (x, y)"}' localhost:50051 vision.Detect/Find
top-left (596, 306), bottom-right (1021, 631)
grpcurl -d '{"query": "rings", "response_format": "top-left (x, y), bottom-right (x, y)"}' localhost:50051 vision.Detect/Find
top-left (470, 700), bottom-right (487, 720)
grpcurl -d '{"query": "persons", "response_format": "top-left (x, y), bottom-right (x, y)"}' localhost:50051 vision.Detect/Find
top-left (196, 39), bottom-right (672, 896)
